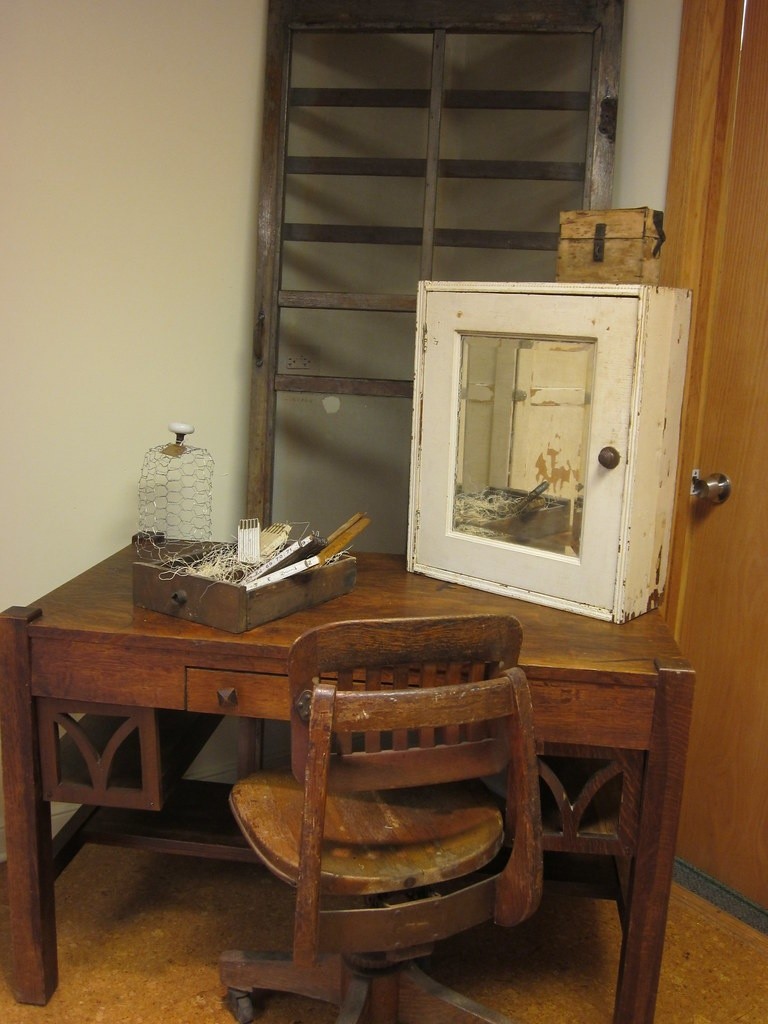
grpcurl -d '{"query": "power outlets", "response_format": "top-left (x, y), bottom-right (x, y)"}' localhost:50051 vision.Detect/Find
top-left (283, 347), bottom-right (321, 370)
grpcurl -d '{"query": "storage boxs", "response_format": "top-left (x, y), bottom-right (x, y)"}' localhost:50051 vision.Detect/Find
top-left (556, 206), bottom-right (664, 286)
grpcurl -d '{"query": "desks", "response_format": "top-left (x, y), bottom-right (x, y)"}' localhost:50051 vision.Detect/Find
top-left (0, 538), bottom-right (698, 1024)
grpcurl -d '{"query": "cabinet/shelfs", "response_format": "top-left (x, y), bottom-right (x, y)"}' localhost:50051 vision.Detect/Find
top-left (407, 279), bottom-right (693, 626)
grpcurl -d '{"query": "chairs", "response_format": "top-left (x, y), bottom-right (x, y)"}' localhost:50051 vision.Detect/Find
top-left (219, 610), bottom-right (546, 1024)
